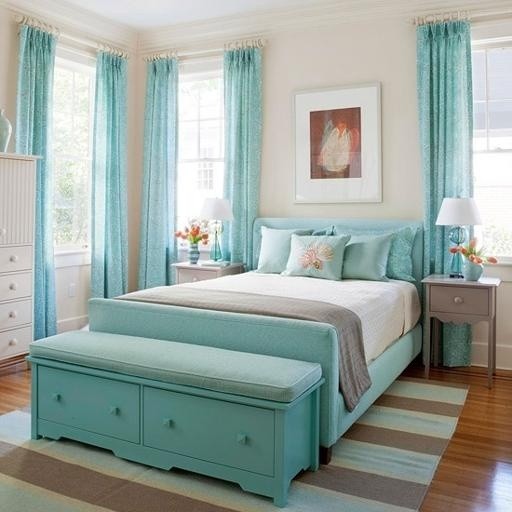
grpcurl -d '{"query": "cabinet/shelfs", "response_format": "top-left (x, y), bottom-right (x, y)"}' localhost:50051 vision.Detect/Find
top-left (170, 259), bottom-right (244, 284)
top-left (1, 153), bottom-right (43, 374)
top-left (29, 328), bottom-right (325, 508)
top-left (421, 273), bottom-right (502, 392)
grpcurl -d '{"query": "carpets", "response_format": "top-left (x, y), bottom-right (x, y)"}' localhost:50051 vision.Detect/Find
top-left (0, 367), bottom-right (471, 512)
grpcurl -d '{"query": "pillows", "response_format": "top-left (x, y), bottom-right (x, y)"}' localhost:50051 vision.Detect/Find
top-left (328, 224), bottom-right (418, 281)
top-left (281, 233), bottom-right (351, 281)
top-left (256, 226), bottom-right (314, 273)
top-left (342, 234), bottom-right (393, 280)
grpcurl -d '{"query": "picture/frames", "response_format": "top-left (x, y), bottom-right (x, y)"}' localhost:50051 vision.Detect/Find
top-left (292, 83), bottom-right (382, 203)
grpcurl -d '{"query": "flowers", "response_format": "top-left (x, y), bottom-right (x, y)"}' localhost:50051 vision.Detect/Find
top-left (175, 218), bottom-right (208, 244)
top-left (451, 238), bottom-right (498, 264)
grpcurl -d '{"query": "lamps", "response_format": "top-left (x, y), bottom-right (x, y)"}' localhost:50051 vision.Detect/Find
top-left (198, 197), bottom-right (232, 262)
top-left (432, 197), bottom-right (484, 278)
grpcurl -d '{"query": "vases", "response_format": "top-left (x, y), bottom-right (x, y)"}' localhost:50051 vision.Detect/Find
top-left (0, 108), bottom-right (13, 153)
top-left (187, 243), bottom-right (203, 265)
top-left (461, 259), bottom-right (484, 282)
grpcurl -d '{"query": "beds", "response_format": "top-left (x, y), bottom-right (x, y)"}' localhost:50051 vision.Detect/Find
top-left (86, 215), bottom-right (423, 469)
